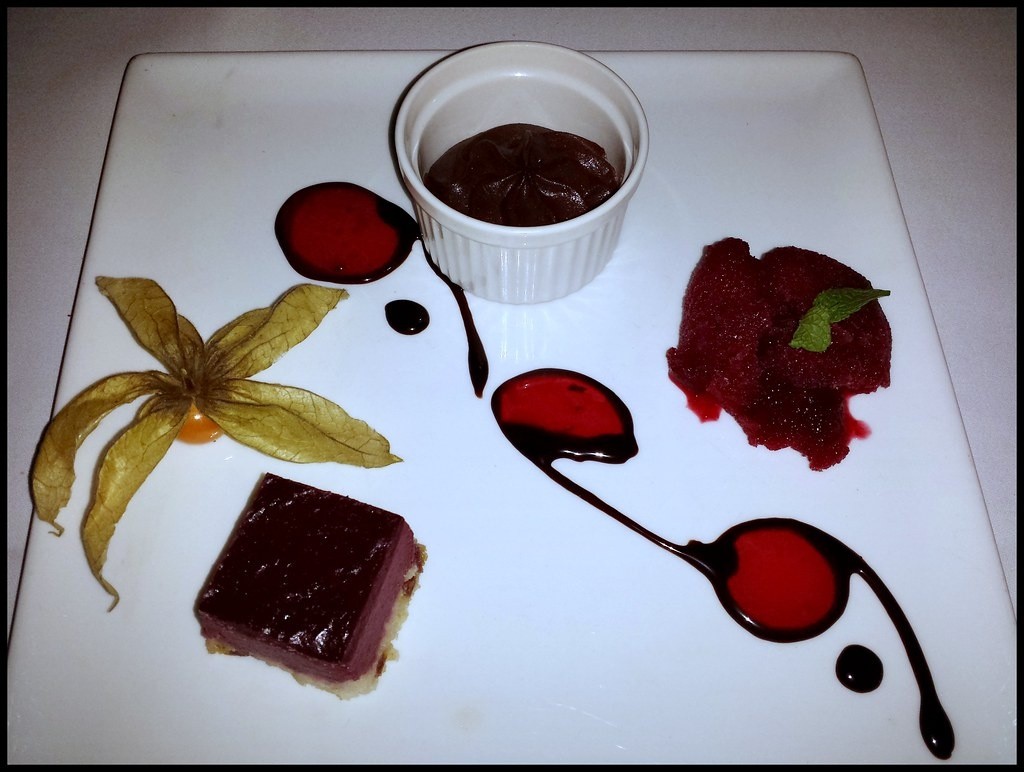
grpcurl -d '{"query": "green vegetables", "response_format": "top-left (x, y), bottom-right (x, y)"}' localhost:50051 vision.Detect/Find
top-left (31, 277), bottom-right (406, 612)
top-left (789, 287), bottom-right (891, 352)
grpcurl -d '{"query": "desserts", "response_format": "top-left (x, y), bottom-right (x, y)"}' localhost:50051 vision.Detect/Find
top-left (194, 474), bottom-right (426, 703)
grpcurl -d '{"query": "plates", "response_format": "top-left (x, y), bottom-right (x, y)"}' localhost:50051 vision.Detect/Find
top-left (8, 50), bottom-right (1016, 767)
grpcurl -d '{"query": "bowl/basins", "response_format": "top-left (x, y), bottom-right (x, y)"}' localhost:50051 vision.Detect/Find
top-left (392, 40), bottom-right (648, 303)
